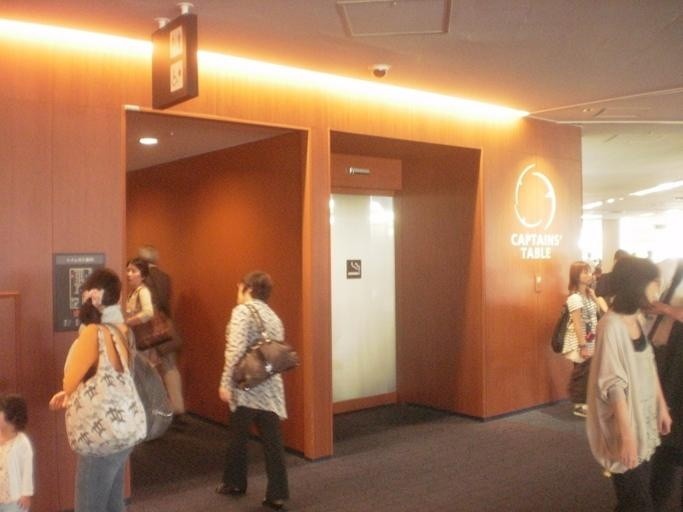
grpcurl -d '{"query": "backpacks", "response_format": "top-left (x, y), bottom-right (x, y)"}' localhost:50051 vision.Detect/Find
top-left (104, 323), bottom-right (174, 443)
top-left (550, 291), bottom-right (581, 354)
top-left (230, 303), bottom-right (301, 393)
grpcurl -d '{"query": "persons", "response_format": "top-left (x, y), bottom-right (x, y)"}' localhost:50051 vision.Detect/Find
top-left (138, 246), bottom-right (188, 431)
top-left (125, 256), bottom-right (163, 369)
top-left (0, 392), bottom-right (35, 511)
top-left (562, 249), bottom-right (682, 512)
top-left (214, 267), bottom-right (290, 511)
top-left (46, 267), bottom-right (138, 511)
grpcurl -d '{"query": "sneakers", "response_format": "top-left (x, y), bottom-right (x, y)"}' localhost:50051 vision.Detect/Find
top-left (573, 403), bottom-right (587, 418)
top-left (215, 481), bottom-right (246, 495)
top-left (261, 497), bottom-right (285, 511)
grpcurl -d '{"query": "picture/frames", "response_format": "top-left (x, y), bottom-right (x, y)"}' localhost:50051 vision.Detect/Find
top-left (51, 253), bottom-right (106, 332)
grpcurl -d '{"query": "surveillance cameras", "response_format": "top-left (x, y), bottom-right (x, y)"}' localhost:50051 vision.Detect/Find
top-left (369, 64), bottom-right (390, 78)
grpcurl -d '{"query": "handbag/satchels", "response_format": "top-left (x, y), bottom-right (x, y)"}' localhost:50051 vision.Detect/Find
top-left (154, 323), bottom-right (185, 358)
top-left (64, 323), bottom-right (149, 460)
top-left (124, 286), bottom-right (175, 352)
